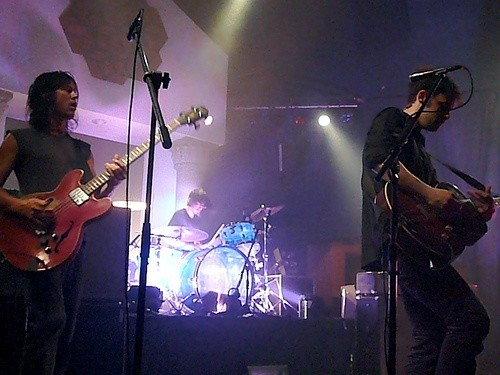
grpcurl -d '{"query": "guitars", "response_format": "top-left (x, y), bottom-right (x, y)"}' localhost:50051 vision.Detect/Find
top-left (0, 103), bottom-right (210, 273)
top-left (375, 179), bottom-right (500, 270)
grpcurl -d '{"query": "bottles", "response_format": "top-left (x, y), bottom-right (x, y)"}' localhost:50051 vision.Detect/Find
top-left (299, 295), bottom-right (312, 320)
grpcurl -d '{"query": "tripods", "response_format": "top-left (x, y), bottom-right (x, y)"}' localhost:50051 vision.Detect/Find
top-left (246, 213), bottom-right (301, 319)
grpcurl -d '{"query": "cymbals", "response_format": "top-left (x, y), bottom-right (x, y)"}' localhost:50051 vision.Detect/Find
top-left (151, 227), bottom-right (209, 243)
top-left (248, 204), bottom-right (286, 224)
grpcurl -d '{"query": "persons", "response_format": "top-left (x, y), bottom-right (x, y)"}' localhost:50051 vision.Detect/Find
top-left (361, 67), bottom-right (496, 375)
top-left (169, 188), bottom-right (211, 260)
top-left (0, 71), bottom-right (127, 375)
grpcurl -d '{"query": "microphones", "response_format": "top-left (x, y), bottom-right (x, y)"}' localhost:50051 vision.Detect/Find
top-left (409, 64), bottom-right (463, 82)
top-left (127, 9), bottom-right (144, 42)
top-left (229, 288), bottom-right (240, 298)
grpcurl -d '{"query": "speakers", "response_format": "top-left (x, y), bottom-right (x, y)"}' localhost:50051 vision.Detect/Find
top-left (64, 206), bottom-right (131, 375)
top-left (351, 270), bottom-right (413, 375)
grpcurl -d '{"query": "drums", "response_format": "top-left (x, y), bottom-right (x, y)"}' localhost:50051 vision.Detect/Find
top-left (219, 222), bottom-right (262, 258)
top-left (178, 246), bottom-right (255, 313)
